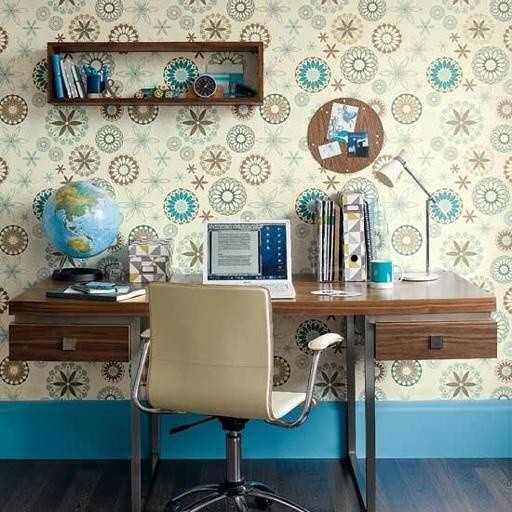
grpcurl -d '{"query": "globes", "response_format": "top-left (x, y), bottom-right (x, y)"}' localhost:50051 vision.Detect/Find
top-left (41, 182), bottom-right (119, 282)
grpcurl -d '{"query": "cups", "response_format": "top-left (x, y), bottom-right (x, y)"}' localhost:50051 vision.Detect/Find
top-left (369, 259), bottom-right (404, 289)
top-left (87, 74), bottom-right (106, 98)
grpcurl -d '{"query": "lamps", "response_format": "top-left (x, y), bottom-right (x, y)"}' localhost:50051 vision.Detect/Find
top-left (376, 148), bottom-right (446, 282)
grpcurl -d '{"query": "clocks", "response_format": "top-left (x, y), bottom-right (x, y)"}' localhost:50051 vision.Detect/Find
top-left (194, 73), bottom-right (217, 99)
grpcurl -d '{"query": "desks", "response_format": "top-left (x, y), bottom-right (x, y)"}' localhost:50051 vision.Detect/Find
top-left (5, 269), bottom-right (498, 511)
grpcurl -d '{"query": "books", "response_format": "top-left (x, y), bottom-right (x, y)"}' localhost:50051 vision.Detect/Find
top-left (315, 192), bottom-right (374, 283)
top-left (45, 282), bottom-right (146, 303)
top-left (52, 55), bottom-right (85, 98)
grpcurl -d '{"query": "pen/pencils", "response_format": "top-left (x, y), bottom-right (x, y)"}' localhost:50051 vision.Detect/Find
top-left (85, 290), bottom-right (115, 294)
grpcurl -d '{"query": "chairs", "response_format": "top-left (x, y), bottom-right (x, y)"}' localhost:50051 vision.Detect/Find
top-left (133, 282), bottom-right (345, 512)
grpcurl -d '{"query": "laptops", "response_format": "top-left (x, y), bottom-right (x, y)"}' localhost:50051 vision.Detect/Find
top-left (203, 219), bottom-right (296, 302)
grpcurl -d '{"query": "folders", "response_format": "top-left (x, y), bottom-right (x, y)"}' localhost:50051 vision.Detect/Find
top-left (343, 199), bottom-right (368, 281)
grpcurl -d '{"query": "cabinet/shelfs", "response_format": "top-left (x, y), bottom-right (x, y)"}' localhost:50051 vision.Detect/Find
top-left (47, 42), bottom-right (264, 107)
